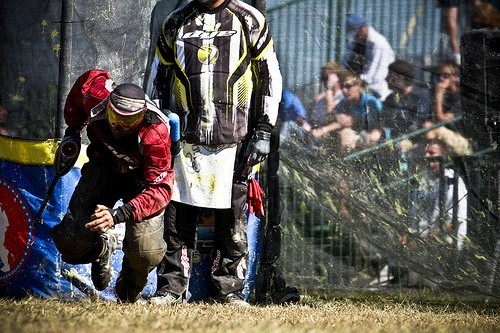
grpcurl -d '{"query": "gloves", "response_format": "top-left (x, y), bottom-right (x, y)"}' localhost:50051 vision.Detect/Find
top-left (244, 131), bottom-right (272, 167)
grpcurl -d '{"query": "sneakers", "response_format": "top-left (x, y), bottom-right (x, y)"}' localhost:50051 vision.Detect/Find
top-left (91, 232), bottom-right (118, 291)
top-left (220, 292), bottom-right (250, 306)
top-left (148, 292), bottom-right (183, 306)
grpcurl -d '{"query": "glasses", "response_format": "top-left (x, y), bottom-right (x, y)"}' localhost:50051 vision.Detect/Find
top-left (436, 72), bottom-right (454, 79)
top-left (339, 83), bottom-right (357, 89)
top-left (106, 97), bottom-right (147, 131)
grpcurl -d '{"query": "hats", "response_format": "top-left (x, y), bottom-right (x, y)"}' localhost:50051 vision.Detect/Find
top-left (347, 14), bottom-right (365, 31)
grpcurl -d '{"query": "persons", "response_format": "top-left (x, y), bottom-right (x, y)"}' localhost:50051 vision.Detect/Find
top-left (53, 68), bottom-right (175, 303)
top-left (147, 0), bottom-right (283, 305)
top-left (279, 0), bottom-right (500, 290)
